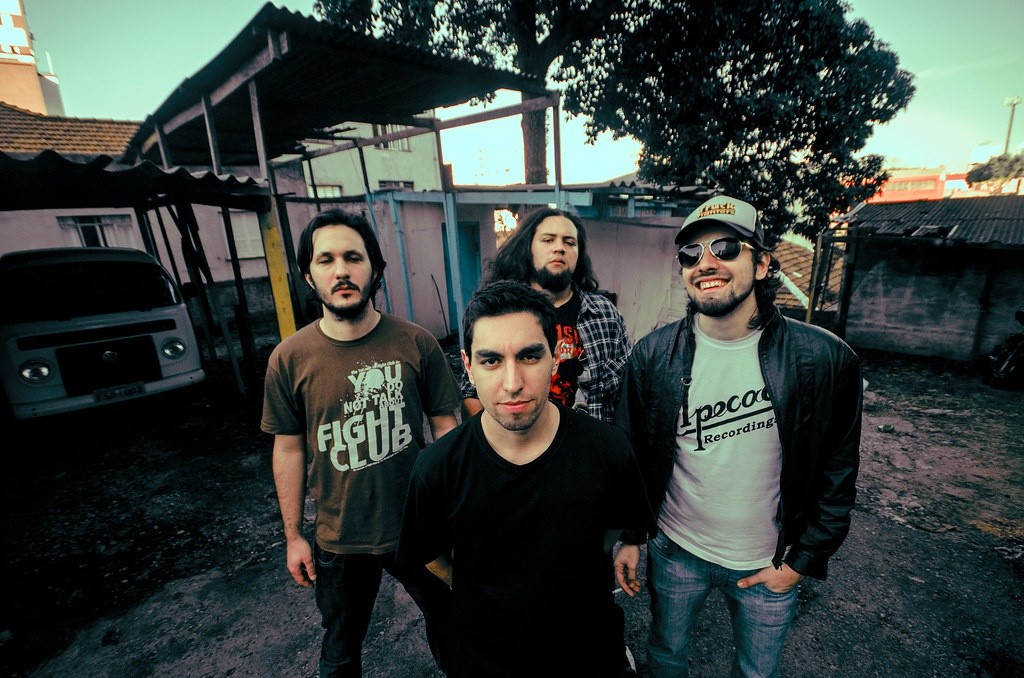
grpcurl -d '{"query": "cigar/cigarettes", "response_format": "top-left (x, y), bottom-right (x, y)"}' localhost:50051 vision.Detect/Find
top-left (612, 583), bottom-right (634, 593)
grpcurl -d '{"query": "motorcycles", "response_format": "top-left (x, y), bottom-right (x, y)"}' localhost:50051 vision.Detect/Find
top-left (988, 306), bottom-right (1024, 391)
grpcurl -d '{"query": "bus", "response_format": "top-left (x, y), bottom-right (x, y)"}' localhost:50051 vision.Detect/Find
top-left (1, 246), bottom-right (208, 435)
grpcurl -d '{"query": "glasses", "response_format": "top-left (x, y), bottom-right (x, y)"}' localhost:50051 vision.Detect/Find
top-left (674, 235), bottom-right (756, 269)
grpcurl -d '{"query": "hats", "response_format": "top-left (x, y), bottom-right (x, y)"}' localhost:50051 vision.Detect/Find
top-left (674, 195), bottom-right (766, 245)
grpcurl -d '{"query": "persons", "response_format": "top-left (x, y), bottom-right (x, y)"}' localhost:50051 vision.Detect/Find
top-left (614, 197), bottom-right (864, 678)
top-left (397, 281), bottom-right (658, 678)
top-left (462, 203), bottom-right (632, 426)
top-left (259, 205), bottom-right (465, 678)
